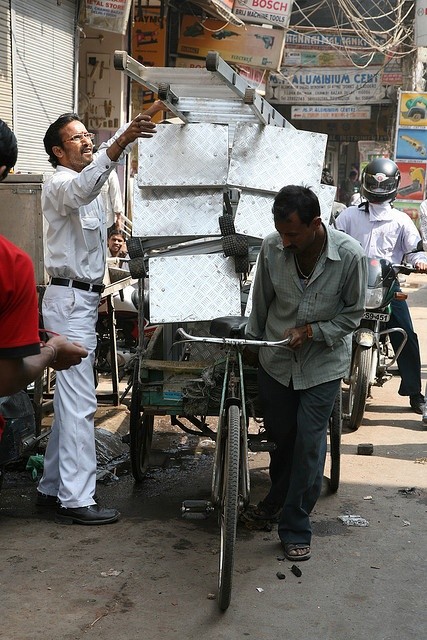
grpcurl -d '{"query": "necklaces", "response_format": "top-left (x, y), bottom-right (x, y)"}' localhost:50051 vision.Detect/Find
top-left (295, 229), bottom-right (327, 280)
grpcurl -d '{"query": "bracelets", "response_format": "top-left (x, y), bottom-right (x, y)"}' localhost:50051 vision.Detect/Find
top-left (307, 324), bottom-right (314, 343)
top-left (115, 139), bottom-right (124, 150)
top-left (43, 343), bottom-right (57, 367)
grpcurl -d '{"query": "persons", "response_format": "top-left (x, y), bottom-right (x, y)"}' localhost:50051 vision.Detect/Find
top-left (418, 197), bottom-right (427, 424)
top-left (330, 156), bottom-right (425, 418)
top-left (37, 111), bottom-right (159, 525)
top-left (93, 168), bottom-right (123, 233)
top-left (241, 185), bottom-right (365, 561)
top-left (2, 119), bottom-right (89, 398)
top-left (103, 230), bottom-right (130, 287)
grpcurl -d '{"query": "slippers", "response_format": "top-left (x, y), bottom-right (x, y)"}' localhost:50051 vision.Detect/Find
top-left (253, 501), bottom-right (283, 520)
top-left (282, 541), bottom-right (312, 561)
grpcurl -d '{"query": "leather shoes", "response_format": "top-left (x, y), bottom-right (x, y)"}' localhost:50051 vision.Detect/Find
top-left (52, 501), bottom-right (119, 526)
top-left (35, 491), bottom-right (101, 514)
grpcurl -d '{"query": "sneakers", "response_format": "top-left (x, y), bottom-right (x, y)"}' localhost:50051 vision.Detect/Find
top-left (411, 395), bottom-right (425, 415)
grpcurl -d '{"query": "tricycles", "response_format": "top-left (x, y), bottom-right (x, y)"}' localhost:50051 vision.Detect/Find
top-left (122, 316), bottom-right (350, 611)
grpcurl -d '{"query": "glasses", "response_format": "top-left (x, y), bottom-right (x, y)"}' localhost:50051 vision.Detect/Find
top-left (51, 132), bottom-right (95, 146)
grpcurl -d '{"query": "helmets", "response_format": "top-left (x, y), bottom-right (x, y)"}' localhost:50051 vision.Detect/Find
top-left (359, 159), bottom-right (401, 204)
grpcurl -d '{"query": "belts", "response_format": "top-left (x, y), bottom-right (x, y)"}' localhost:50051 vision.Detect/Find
top-left (47, 275), bottom-right (106, 295)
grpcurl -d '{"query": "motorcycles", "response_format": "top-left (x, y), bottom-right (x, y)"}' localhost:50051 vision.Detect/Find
top-left (338, 230), bottom-right (427, 430)
